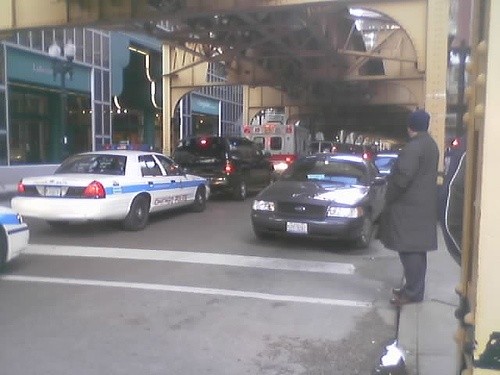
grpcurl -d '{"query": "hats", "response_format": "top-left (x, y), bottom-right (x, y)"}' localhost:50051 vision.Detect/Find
top-left (407, 110), bottom-right (430, 132)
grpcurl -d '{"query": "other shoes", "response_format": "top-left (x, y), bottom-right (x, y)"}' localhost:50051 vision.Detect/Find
top-left (389, 287), bottom-right (423, 306)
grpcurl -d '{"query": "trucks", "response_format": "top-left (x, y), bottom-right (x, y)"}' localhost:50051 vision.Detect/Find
top-left (245, 123), bottom-right (313, 177)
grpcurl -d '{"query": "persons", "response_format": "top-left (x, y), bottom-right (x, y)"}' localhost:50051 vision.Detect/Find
top-left (371, 110), bottom-right (439, 305)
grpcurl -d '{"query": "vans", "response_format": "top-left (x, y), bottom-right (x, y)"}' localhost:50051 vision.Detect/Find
top-left (174, 135), bottom-right (275, 199)
top-left (311, 140), bottom-right (342, 154)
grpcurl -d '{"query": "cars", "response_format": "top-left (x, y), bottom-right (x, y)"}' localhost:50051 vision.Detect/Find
top-left (1, 207), bottom-right (32, 269)
top-left (11, 143), bottom-right (211, 231)
top-left (250, 149), bottom-right (403, 250)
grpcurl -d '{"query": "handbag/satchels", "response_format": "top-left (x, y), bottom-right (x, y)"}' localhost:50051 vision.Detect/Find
top-left (477, 331), bottom-right (499, 368)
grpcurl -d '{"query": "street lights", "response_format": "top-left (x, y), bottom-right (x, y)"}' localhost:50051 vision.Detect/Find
top-left (48, 40), bottom-right (75, 162)
